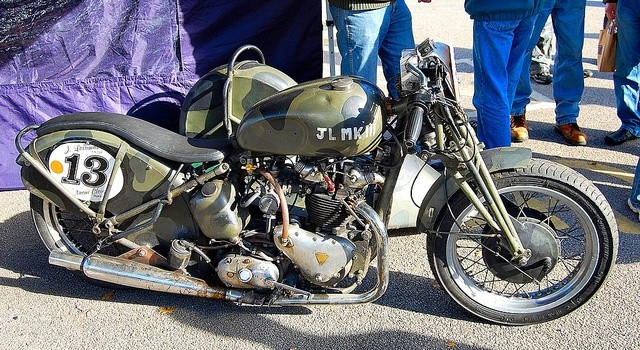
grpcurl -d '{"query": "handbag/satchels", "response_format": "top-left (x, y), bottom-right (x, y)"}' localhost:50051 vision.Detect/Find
top-left (597, 18), bottom-right (616, 72)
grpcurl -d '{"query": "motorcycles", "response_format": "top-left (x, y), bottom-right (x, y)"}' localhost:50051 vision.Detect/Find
top-left (15, 40), bottom-right (619, 327)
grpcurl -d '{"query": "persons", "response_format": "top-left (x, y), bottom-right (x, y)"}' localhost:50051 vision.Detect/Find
top-left (596, 1), bottom-right (640, 145)
top-left (327, 0), bottom-right (421, 100)
top-left (464, 1), bottom-right (530, 149)
top-left (511, 1), bottom-right (589, 145)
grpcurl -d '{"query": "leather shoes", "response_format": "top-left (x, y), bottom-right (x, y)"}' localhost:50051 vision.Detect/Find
top-left (604, 129), bottom-right (635, 144)
top-left (555, 122), bottom-right (588, 145)
top-left (510, 114), bottom-right (529, 141)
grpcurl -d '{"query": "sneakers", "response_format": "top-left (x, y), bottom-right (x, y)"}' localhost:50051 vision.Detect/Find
top-left (531, 72), bottom-right (553, 84)
